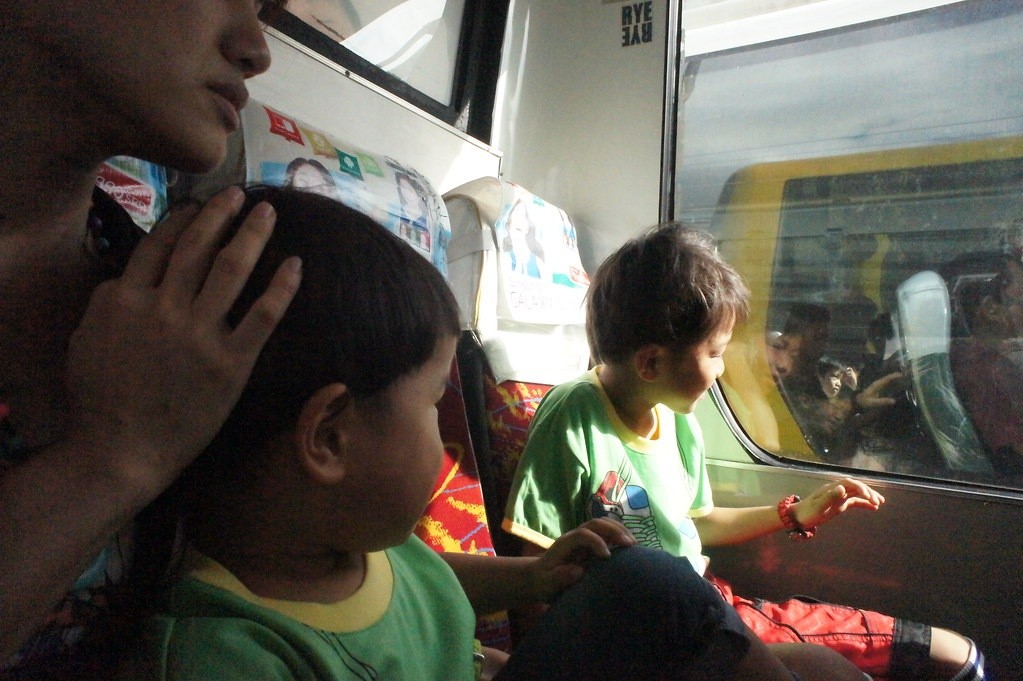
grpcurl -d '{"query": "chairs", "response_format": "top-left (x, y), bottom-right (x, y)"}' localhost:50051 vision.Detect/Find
top-left (94, 103), bottom-right (602, 656)
top-left (885, 269), bottom-right (995, 473)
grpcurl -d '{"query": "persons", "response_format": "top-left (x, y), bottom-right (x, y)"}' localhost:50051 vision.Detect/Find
top-left (501, 221), bottom-right (1000, 680)
top-left (965, 244), bottom-right (1023, 484)
top-left (721, 293), bottom-right (802, 452)
top-left (765, 295), bottom-right (905, 435)
top-left (76, 176), bottom-right (876, 680)
top-left (0, 0), bottom-right (301, 681)
top-left (818, 356), bottom-right (861, 400)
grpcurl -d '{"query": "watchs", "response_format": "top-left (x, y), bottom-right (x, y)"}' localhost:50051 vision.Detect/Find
top-left (778, 494), bottom-right (818, 544)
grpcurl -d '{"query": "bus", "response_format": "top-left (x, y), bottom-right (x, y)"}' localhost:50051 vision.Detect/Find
top-left (678, 1), bottom-right (1023, 490)
